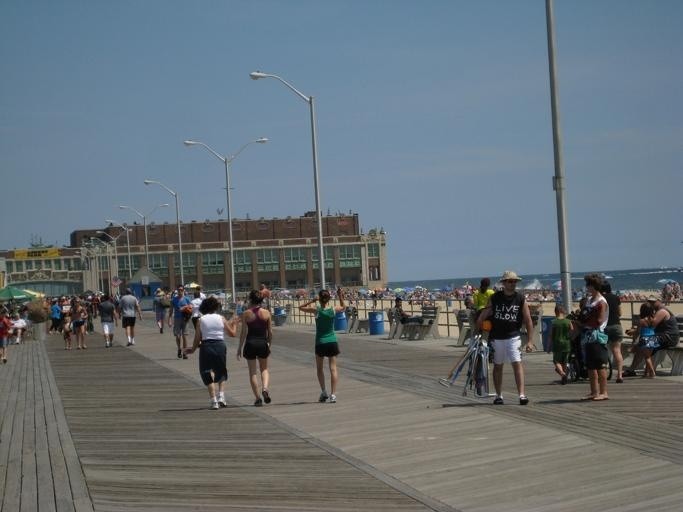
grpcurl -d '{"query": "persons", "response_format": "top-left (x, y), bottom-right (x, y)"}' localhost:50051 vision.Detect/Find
top-left (46, 288), bottom-right (142, 351)
top-left (454, 271), bottom-right (682, 405)
top-left (0, 303), bottom-right (28, 363)
top-left (185, 297), bottom-right (242, 409)
top-left (299, 287), bottom-right (425, 404)
top-left (152, 284), bottom-right (207, 360)
top-left (236, 284), bottom-right (274, 406)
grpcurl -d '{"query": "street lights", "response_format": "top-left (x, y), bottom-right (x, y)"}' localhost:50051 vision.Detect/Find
top-left (184, 138), bottom-right (269, 308)
top-left (144, 178), bottom-right (186, 306)
top-left (74, 231), bottom-right (123, 299)
top-left (118, 203), bottom-right (168, 298)
top-left (248, 71), bottom-right (325, 293)
top-left (105, 220), bottom-right (132, 283)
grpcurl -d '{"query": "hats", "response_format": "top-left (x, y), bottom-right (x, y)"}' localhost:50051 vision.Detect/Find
top-left (153, 288), bottom-right (164, 296)
top-left (647, 294), bottom-right (661, 302)
top-left (499, 271), bottom-right (522, 282)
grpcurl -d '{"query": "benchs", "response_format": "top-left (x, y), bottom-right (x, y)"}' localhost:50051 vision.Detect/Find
top-left (454, 309), bottom-right (476, 345)
top-left (344, 307), bottom-right (369, 334)
top-left (520, 304), bottom-right (545, 352)
top-left (387, 307), bottom-right (403, 340)
top-left (421, 306), bottom-right (442, 339)
top-left (280, 304), bottom-right (294, 326)
top-left (618, 314), bottom-right (683, 374)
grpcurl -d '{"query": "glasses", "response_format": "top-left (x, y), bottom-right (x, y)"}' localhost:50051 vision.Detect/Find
top-left (507, 280), bottom-right (516, 283)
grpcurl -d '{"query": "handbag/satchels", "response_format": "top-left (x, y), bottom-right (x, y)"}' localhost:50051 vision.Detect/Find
top-left (584, 343), bottom-right (607, 369)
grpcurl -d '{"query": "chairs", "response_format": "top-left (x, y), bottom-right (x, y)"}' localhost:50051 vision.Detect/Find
top-left (6, 332), bottom-right (25, 344)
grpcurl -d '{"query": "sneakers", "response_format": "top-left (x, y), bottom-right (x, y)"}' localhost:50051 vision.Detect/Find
top-left (177, 350), bottom-right (181, 358)
top-left (615, 379), bottom-right (622, 382)
top-left (182, 354), bottom-right (187, 359)
top-left (493, 398), bottom-right (502, 404)
top-left (261, 388), bottom-right (270, 404)
top-left (208, 402), bottom-right (218, 410)
top-left (254, 400), bottom-right (261, 406)
top-left (519, 397), bottom-right (527, 404)
top-left (561, 375), bottom-right (567, 385)
top-left (217, 396), bottom-right (226, 407)
top-left (329, 395), bottom-right (336, 403)
top-left (318, 393), bottom-right (328, 402)
top-left (621, 370), bottom-right (635, 377)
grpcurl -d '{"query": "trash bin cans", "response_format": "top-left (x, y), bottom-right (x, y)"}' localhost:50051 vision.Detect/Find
top-left (274, 307), bottom-right (284, 315)
top-left (540, 316), bottom-right (558, 351)
top-left (334, 311), bottom-right (348, 330)
top-left (368, 312), bottom-right (384, 335)
